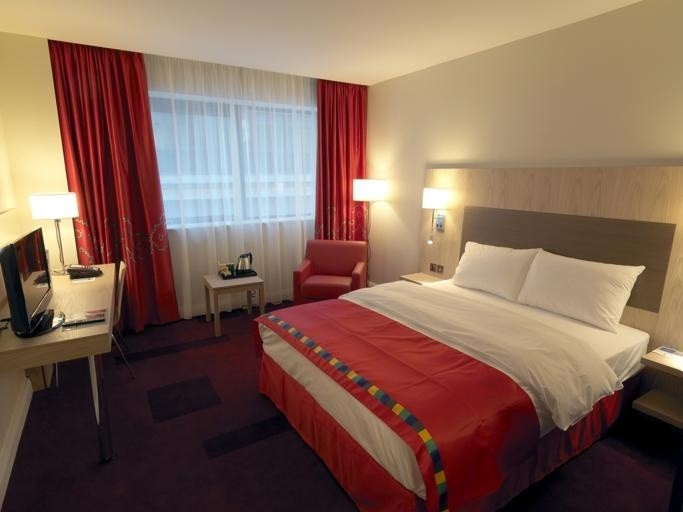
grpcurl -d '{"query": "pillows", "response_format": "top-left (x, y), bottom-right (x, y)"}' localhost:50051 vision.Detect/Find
top-left (449, 240), bottom-right (646, 334)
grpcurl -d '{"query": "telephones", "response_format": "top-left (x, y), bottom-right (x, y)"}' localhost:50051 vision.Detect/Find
top-left (67, 264), bottom-right (100, 279)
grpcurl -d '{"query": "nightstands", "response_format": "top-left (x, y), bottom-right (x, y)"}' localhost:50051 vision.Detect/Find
top-left (399, 272), bottom-right (444, 285)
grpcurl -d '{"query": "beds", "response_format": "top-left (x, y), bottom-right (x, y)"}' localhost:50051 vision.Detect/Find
top-left (258, 167), bottom-right (682, 512)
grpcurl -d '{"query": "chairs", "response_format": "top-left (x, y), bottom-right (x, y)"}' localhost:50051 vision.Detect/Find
top-left (54, 261), bottom-right (135, 392)
top-left (292, 239), bottom-right (367, 306)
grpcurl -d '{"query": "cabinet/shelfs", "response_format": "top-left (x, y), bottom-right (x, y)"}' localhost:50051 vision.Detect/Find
top-left (631, 345), bottom-right (682, 429)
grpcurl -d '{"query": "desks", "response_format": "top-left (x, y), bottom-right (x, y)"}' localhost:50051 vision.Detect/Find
top-left (202, 274), bottom-right (264, 338)
top-left (0, 263), bottom-right (118, 464)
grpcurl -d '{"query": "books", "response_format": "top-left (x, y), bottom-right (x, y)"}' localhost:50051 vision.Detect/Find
top-left (61, 308), bottom-right (105, 326)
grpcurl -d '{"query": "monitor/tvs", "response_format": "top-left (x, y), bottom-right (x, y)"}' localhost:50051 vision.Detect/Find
top-left (0, 227), bottom-right (65, 339)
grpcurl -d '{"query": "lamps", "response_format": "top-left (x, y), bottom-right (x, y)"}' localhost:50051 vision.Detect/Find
top-left (421, 188), bottom-right (451, 232)
top-left (352, 178), bottom-right (387, 242)
top-left (29, 191), bottom-right (78, 276)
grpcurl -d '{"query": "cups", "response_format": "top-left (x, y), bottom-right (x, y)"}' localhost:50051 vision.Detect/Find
top-left (225, 263), bottom-right (234, 274)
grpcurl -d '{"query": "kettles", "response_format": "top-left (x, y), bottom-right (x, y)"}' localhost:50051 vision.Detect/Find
top-left (235, 252), bottom-right (252, 275)
